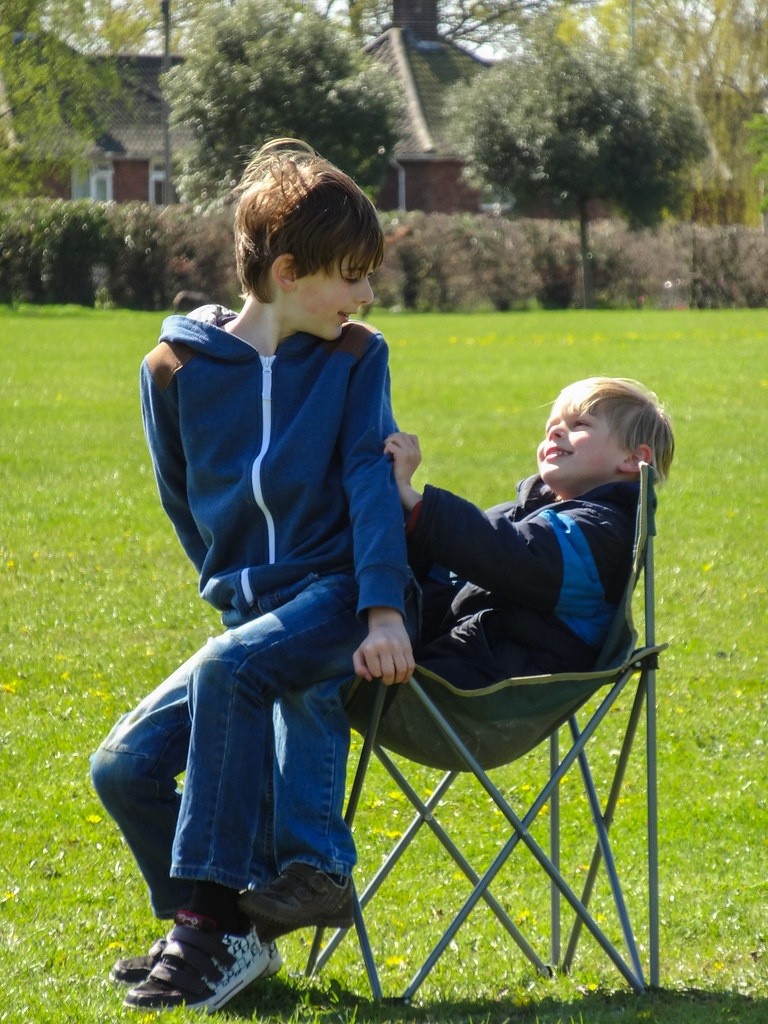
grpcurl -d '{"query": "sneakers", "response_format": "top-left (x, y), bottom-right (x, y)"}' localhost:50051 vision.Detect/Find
top-left (111, 934), bottom-right (283, 986)
top-left (122, 920), bottom-right (269, 1012)
top-left (237, 861), bottom-right (357, 929)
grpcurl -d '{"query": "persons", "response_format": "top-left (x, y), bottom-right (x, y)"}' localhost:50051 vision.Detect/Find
top-left (89, 136), bottom-right (421, 1014)
top-left (238, 376), bottom-right (674, 943)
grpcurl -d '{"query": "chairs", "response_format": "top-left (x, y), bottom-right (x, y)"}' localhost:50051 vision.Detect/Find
top-left (303, 460), bottom-right (667, 1013)
